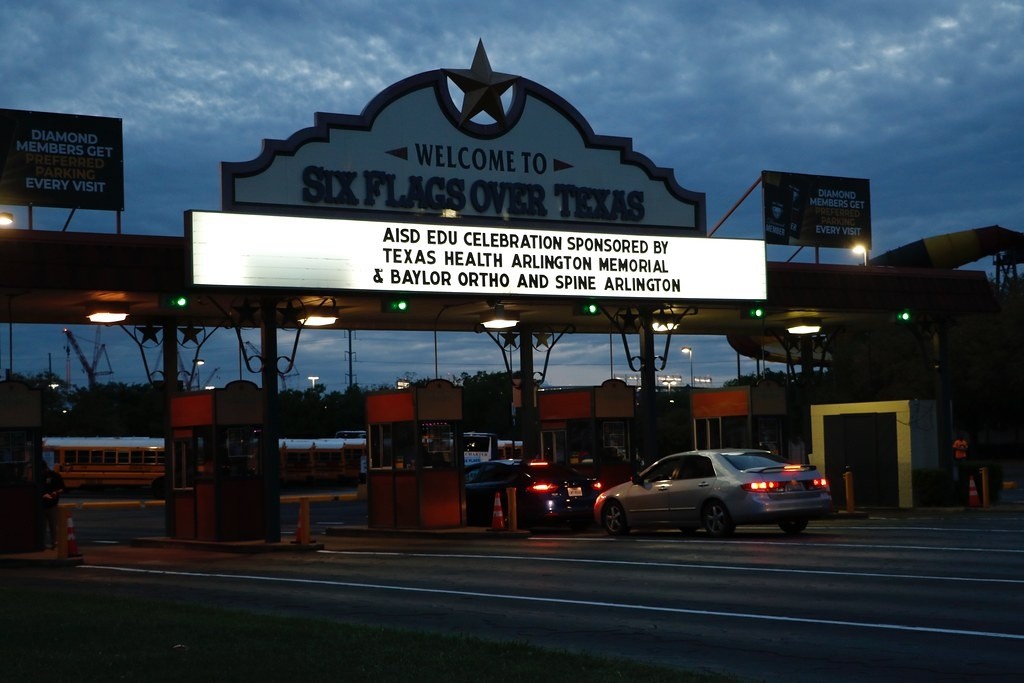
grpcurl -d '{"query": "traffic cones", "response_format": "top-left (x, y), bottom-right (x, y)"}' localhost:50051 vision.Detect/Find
top-left (965, 474), bottom-right (984, 507)
top-left (489, 490), bottom-right (507, 529)
top-left (291, 499), bottom-right (307, 543)
top-left (65, 512), bottom-right (82, 556)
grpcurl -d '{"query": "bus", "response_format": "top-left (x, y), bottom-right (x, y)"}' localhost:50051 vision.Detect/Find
top-left (278, 438), bottom-right (367, 486)
top-left (447, 433), bottom-right (523, 466)
top-left (41, 436), bottom-right (166, 501)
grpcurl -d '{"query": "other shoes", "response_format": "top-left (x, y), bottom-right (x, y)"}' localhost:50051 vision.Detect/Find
top-left (47, 542), bottom-right (56, 549)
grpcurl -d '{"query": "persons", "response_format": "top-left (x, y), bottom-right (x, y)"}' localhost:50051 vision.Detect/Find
top-left (952, 432), bottom-right (968, 466)
top-left (41, 466), bottom-right (65, 552)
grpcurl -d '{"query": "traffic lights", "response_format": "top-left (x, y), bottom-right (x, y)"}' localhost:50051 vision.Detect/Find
top-left (381, 298), bottom-right (409, 313)
top-left (162, 295), bottom-right (192, 311)
top-left (894, 308), bottom-right (914, 322)
top-left (740, 305), bottom-right (766, 320)
top-left (577, 303), bottom-right (598, 315)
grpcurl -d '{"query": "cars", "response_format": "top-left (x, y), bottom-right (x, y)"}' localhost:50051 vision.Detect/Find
top-left (590, 446), bottom-right (836, 536)
top-left (464, 457), bottom-right (604, 528)
